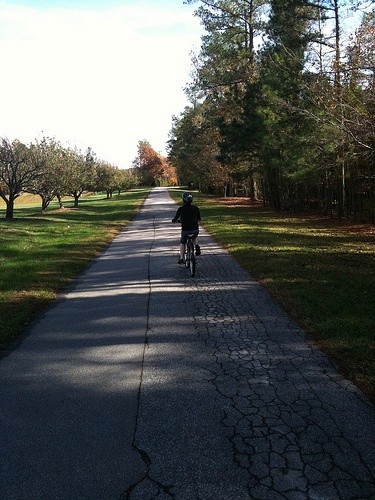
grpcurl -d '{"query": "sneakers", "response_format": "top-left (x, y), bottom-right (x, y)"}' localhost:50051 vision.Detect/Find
top-left (177, 259), bottom-right (184, 264)
top-left (195, 244), bottom-right (201, 256)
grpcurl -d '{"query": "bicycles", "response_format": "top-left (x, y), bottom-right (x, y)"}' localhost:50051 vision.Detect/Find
top-left (172, 218), bottom-right (202, 277)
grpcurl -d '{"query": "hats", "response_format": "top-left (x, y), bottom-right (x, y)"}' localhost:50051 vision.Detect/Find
top-left (182, 192), bottom-right (193, 202)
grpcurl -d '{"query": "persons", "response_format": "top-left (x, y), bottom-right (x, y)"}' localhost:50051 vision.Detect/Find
top-left (172, 192), bottom-right (201, 264)
